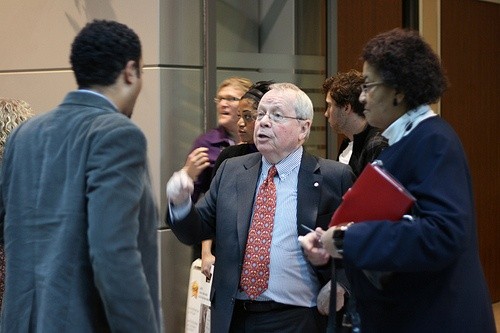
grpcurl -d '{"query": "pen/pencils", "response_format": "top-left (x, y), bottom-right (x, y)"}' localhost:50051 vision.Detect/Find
top-left (300, 223), bottom-right (321, 236)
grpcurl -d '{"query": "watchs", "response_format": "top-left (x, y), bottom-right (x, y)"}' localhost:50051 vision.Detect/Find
top-left (333, 220), bottom-right (354, 256)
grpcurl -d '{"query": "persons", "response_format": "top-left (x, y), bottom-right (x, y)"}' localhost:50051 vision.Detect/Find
top-left (165, 83), bottom-right (357, 332)
top-left (297, 27), bottom-right (498, 333)
top-left (0, 19), bottom-right (161, 333)
top-left (323, 69), bottom-right (383, 177)
top-left (200, 80), bottom-right (277, 279)
top-left (183, 78), bottom-right (254, 258)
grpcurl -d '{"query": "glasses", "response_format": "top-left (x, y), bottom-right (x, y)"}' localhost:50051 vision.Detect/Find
top-left (360, 82), bottom-right (386, 91)
top-left (250, 113), bottom-right (306, 122)
top-left (212, 96), bottom-right (240, 102)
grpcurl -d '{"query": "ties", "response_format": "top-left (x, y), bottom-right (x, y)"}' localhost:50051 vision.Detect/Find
top-left (240, 165), bottom-right (277, 303)
